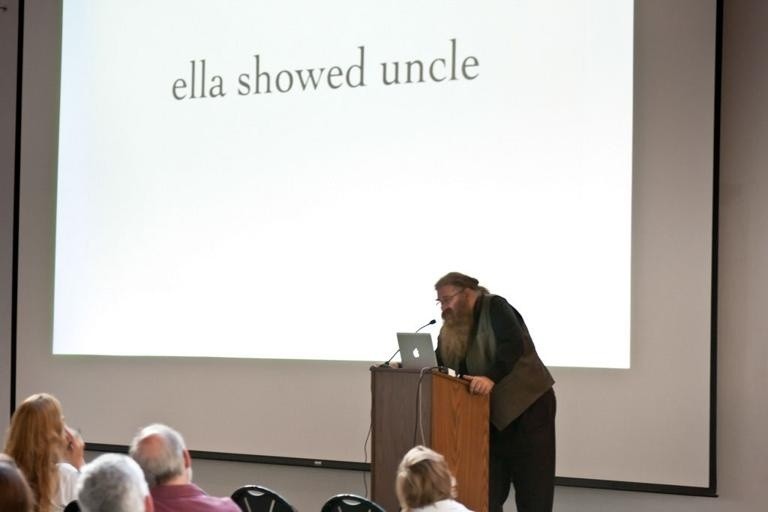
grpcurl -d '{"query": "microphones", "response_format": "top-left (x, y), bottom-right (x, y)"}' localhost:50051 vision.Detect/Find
top-left (378, 316), bottom-right (436, 366)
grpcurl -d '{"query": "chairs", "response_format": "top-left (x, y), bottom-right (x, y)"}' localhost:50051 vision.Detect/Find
top-left (321, 493), bottom-right (387, 512)
top-left (229, 485), bottom-right (299, 511)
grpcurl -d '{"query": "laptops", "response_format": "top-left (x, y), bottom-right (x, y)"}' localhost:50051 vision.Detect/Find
top-left (397, 332), bottom-right (441, 373)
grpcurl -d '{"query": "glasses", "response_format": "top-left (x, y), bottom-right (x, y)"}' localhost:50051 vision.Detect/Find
top-left (436, 290), bottom-right (465, 305)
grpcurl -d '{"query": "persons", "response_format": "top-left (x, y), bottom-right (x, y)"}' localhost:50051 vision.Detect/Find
top-left (393, 443), bottom-right (474, 512)
top-left (128, 423), bottom-right (243, 512)
top-left (0, 452), bottom-right (36, 512)
top-left (74, 451), bottom-right (155, 512)
top-left (3, 392), bottom-right (85, 512)
top-left (370, 271), bottom-right (556, 512)
